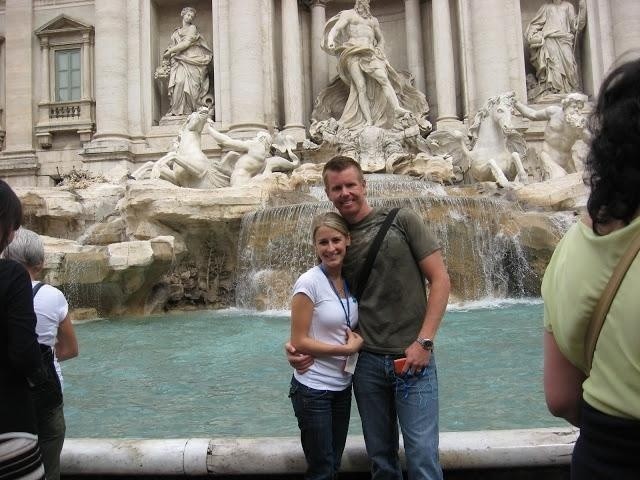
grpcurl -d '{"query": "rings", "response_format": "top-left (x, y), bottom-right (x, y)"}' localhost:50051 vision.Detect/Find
top-left (417, 369), bottom-right (421, 372)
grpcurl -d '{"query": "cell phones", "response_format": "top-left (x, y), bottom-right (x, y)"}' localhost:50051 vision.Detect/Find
top-left (393, 358), bottom-right (407, 375)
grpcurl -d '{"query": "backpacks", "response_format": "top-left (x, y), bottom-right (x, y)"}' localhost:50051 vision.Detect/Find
top-left (30, 346), bottom-right (65, 413)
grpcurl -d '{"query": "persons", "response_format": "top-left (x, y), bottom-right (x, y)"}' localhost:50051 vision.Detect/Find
top-left (510, 92), bottom-right (598, 181)
top-left (154, 7), bottom-right (213, 116)
top-left (523, 0), bottom-right (587, 94)
top-left (320, 0), bottom-right (413, 126)
top-left (0, 180), bottom-right (78, 480)
top-left (540, 60), bottom-right (640, 480)
top-left (321, 156), bottom-right (451, 480)
top-left (286, 212), bottom-right (363, 480)
top-left (205, 119), bottom-right (276, 189)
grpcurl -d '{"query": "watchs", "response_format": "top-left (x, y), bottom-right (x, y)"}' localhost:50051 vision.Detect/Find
top-left (417, 337), bottom-right (434, 351)
